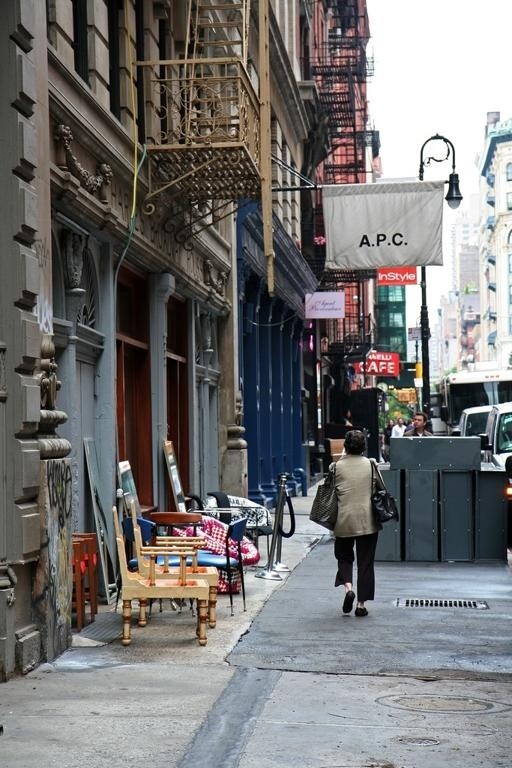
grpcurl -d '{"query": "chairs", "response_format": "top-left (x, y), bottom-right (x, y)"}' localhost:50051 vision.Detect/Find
top-left (72, 488), bottom-right (270, 645)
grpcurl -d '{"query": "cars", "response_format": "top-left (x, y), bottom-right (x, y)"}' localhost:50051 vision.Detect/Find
top-left (447, 401), bottom-right (512, 476)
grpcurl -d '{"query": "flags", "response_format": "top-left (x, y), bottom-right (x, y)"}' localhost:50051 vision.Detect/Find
top-left (320, 180), bottom-right (446, 267)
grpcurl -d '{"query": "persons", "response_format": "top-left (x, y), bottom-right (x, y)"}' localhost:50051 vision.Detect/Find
top-left (383, 414), bottom-right (433, 461)
top-left (324, 430), bottom-right (384, 616)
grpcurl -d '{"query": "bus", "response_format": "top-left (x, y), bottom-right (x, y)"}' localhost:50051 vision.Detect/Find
top-left (421, 367), bottom-right (512, 436)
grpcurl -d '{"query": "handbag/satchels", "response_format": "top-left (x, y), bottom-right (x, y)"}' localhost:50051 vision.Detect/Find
top-left (369, 458), bottom-right (400, 524)
top-left (308, 462), bottom-right (338, 533)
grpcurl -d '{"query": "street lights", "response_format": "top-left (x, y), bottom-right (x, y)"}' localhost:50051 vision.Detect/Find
top-left (418, 130), bottom-right (463, 431)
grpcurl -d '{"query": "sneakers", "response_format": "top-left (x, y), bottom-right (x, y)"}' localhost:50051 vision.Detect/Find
top-left (355, 607), bottom-right (369, 617)
top-left (342, 590), bottom-right (355, 614)
